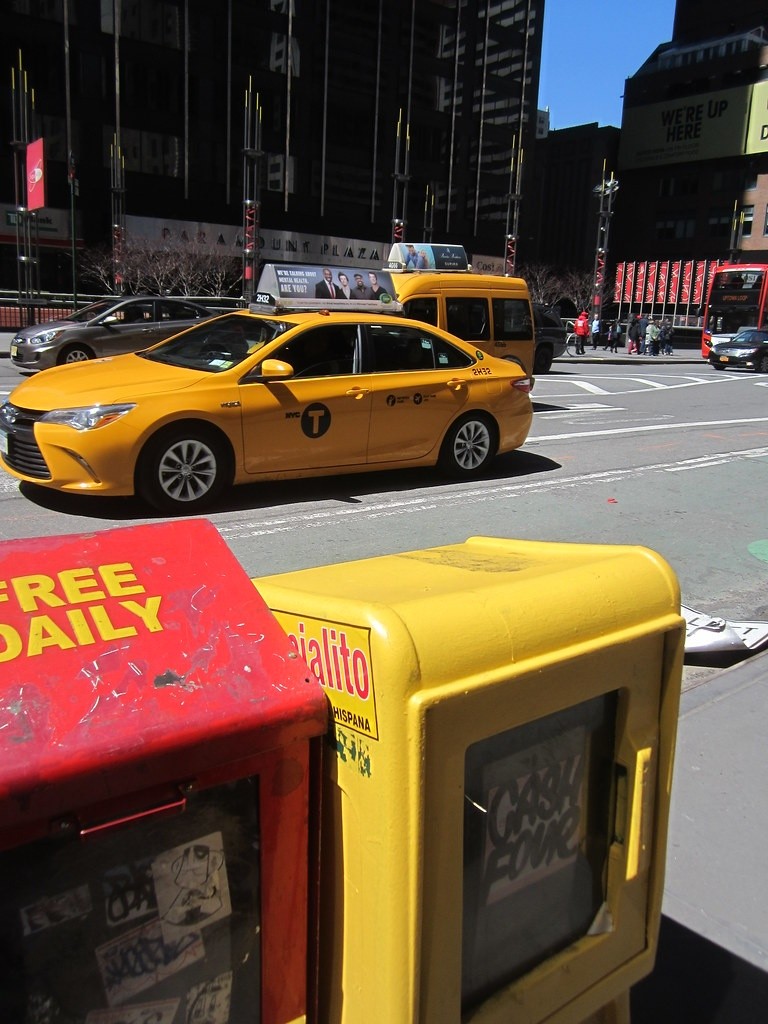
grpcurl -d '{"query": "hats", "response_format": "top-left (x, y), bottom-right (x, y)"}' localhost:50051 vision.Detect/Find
top-left (582, 311), bottom-right (588, 316)
top-left (354, 274), bottom-right (362, 280)
top-left (637, 315), bottom-right (642, 319)
top-left (648, 321), bottom-right (654, 324)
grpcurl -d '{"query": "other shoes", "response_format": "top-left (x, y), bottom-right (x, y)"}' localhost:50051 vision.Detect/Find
top-left (583, 351), bottom-right (585, 354)
top-left (577, 353), bottom-right (581, 355)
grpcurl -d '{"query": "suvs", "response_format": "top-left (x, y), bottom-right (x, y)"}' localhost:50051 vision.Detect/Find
top-left (459, 303), bottom-right (568, 373)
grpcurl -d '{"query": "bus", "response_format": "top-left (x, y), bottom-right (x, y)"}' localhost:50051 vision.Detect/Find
top-left (695, 263), bottom-right (768, 360)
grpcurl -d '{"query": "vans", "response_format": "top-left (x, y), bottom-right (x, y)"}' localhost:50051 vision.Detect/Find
top-left (379, 243), bottom-right (537, 381)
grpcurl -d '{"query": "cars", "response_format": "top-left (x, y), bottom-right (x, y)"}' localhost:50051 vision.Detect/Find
top-left (0, 265), bottom-right (534, 517)
top-left (10, 297), bottom-right (249, 375)
top-left (708, 330), bottom-right (768, 375)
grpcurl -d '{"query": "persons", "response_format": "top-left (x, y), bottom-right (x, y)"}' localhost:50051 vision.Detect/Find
top-left (591, 312), bottom-right (601, 350)
top-left (405, 245), bottom-right (429, 269)
top-left (574, 311), bottom-right (590, 355)
top-left (338, 272), bottom-right (357, 299)
top-left (628, 315), bottom-right (673, 356)
top-left (602, 319), bottom-right (621, 354)
top-left (353, 272), bottom-right (388, 300)
top-left (314, 269), bottom-right (339, 299)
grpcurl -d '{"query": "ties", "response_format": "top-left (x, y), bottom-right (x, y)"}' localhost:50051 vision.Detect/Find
top-left (362, 288), bottom-right (364, 293)
top-left (329, 282), bottom-right (334, 299)
